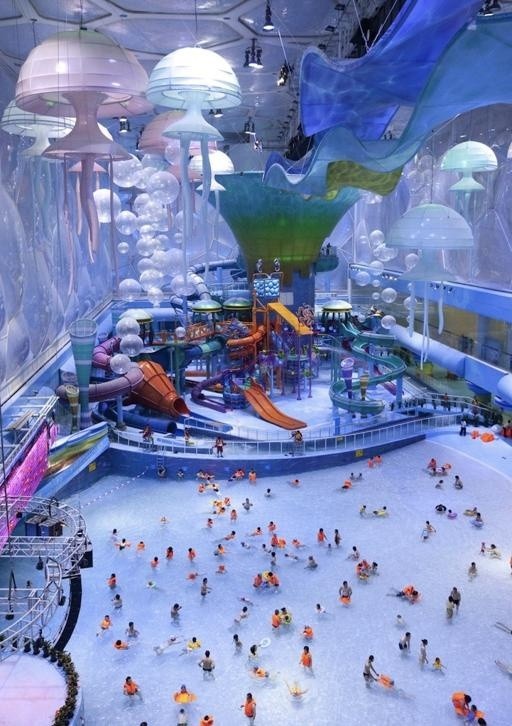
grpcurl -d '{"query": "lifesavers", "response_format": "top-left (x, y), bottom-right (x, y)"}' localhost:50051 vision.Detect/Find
top-left (174, 694), bottom-right (197, 702)
top-left (434, 509), bottom-right (483, 528)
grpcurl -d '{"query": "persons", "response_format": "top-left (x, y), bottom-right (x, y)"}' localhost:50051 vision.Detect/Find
top-left (101, 454), bottom-right (512, 725)
top-left (212, 436), bottom-right (227, 459)
top-left (326, 241), bottom-right (331, 255)
top-left (138, 423), bottom-right (155, 443)
top-left (290, 427), bottom-right (306, 444)
top-left (395, 388), bottom-right (512, 441)
top-left (182, 427), bottom-right (192, 446)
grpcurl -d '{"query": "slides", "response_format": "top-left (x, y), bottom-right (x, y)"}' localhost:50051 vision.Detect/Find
top-left (329, 322), bottom-right (405, 414)
top-left (244, 385), bottom-right (307, 430)
top-left (102, 359), bottom-right (233, 438)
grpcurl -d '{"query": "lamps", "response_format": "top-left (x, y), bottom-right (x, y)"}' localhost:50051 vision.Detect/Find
top-left (284, 1), bottom-right (350, 125)
top-left (242, 4), bottom-right (296, 88)
top-left (475, 0), bottom-right (507, 18)
